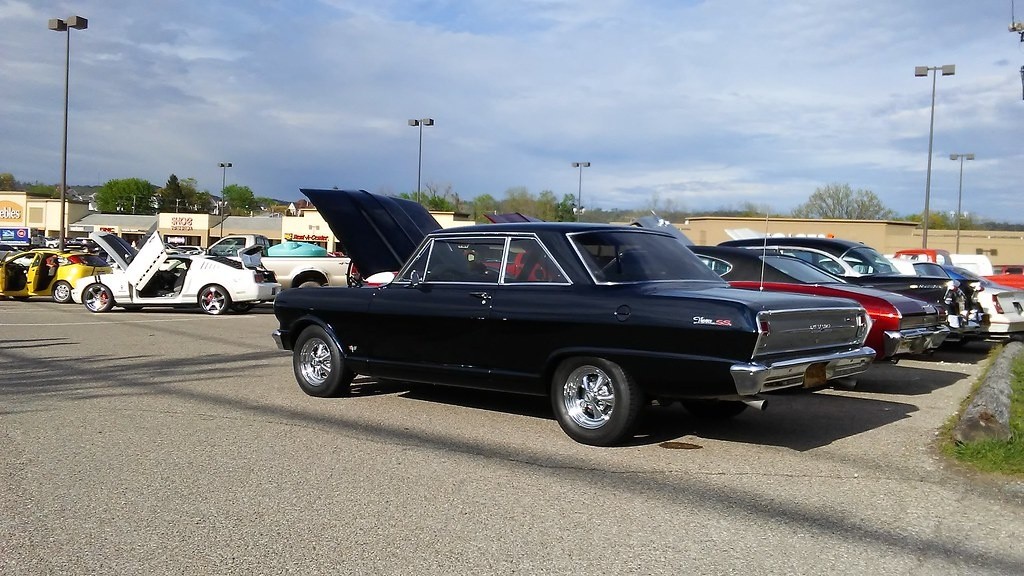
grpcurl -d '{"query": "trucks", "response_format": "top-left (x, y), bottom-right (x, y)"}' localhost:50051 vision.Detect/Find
top-left (0, 227), bottom-right (40, 251)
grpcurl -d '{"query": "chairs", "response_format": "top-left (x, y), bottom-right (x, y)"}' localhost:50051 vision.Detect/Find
top-left (598, 248), bottom-right (651, 282)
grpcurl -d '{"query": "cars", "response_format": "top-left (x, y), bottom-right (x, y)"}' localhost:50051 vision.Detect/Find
top-left (0, 230), bottom-right (398, 315)
top-left (271, 186), bottom-right (877, 446)
top-left (460, 212), bottom-right (1024, 360)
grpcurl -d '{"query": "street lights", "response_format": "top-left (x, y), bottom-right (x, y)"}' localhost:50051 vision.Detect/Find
top-left (217, 163), bottom-right (233, 240)
top-left (572, 161), bottom-right (591, 222)
top-left (48, 14), bottom-right (90, 251)
top-left (914, 64), bottom-right (958, 250)
top-left (408, 117), bottom-right (435, 204)
top-left (949, 153), bottom-right (975, 256)
